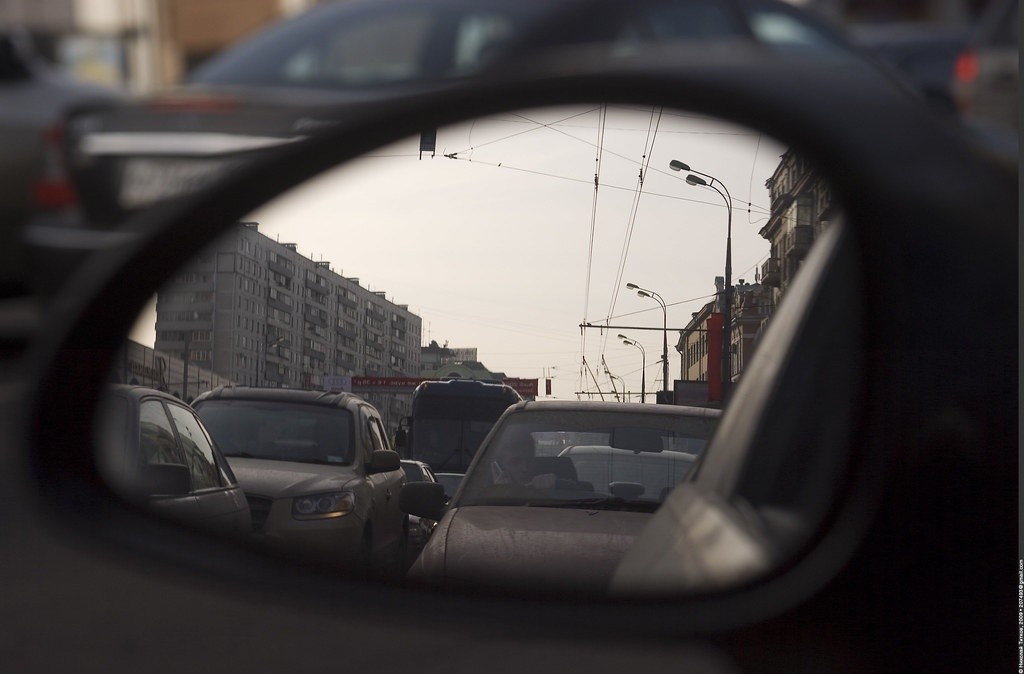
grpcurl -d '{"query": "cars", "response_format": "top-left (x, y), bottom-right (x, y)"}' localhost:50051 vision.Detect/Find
top-left (403, 399), bottom-right (818, 604)
top-left (0, 12), bottom-right (146, 237)
top-left (398, 457), bottom-right (446, 560)
top-left (10, 1), bottom-right (971, 330)
top-left (94, 383), bottom-right (255, 545)
top-left (184, 384), bottom-right (411, 581)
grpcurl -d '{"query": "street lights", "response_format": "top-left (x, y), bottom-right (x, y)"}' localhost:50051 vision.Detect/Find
top-left (616, 333), bottom-right (646, 403)
top-left (669, 158), bottom-right (731, 402)
top-left (604, 368), bottom-right (626, 403)
top-left (625, 282), bottom-right (670, 403)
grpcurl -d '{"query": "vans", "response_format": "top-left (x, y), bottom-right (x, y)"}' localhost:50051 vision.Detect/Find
top-left (396, 377), bottom-right (533, 508)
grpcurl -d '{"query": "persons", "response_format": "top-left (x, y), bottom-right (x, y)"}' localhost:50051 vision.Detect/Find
top-left (422, 430), bottom-right (448, 460)
top-left (489, 426), bottom-right (557, 489)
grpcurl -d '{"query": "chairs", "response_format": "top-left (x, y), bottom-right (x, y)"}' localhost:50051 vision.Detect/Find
top-left (536, 455), bottom-right (581, 481)
